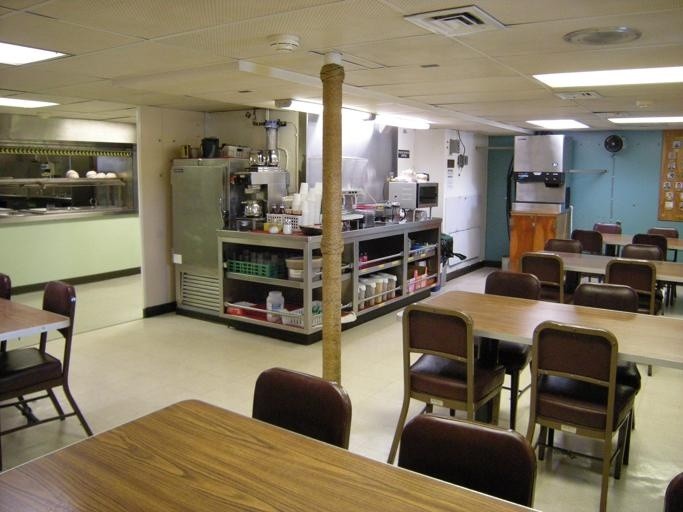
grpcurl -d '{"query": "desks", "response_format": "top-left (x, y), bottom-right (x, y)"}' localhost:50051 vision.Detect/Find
top-left (0, 298), bottom-right (71, 342)
top-left (396, 290), bottom-right (683, 414)
top-left (0, 399), bottom-right (543, 512)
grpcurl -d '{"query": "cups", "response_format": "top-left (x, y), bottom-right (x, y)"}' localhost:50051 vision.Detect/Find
top-left (191, 148), bottom-right (199, 158)
top-left (292, 182), bottom-right (323, 226)
top-left (180, 144), bottom-right (191, 158)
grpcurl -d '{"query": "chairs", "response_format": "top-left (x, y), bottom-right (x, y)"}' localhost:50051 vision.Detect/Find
top-left (385, 303), bottom-right (506, 466)
top-left (474, 270), bottom-right (541, 432)
top-left (252, 368), bottom-right (352, 451)
top-left (524, 320), bottom-right (635, 512)
top-left (398, 413), bottom-right (536, 509)
top-left (663, 472), bottom-right (683, 512)
top-left (0, 280), bottom-right (94, 472)
top-left (524, 222), bottom-right (683, 315)
top-left (574, 283), bottom-right (641, 464)
top-left (0, 273), bottom-right (11, 352)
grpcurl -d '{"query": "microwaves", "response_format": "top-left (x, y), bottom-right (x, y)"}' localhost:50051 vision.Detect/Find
top-left (389, 181), bottom-right (438, 209)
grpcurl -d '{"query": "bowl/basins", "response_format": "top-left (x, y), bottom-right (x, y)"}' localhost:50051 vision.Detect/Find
top-left (299, 225), bottom-right (322, 236)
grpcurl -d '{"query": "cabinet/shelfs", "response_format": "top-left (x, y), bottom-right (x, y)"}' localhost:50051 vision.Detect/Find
top-left (509, 207), bottom-right (572, 273)
top-left (217, 219), bottom-right (447, 346)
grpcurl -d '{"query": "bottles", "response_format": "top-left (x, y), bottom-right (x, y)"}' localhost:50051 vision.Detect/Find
top-left (271, 204), bottom-right (278, 214)
top-left (348, 190), bottom-right (358, 210)
top-left (357, 272), bottom-right (397, 310)
top-left (232, 249), bottom-right (271, 275)
top-left (266, 291), bottom-right (284, 322)
top-left (359, 252), bottom-right (368, 270)
top-left (282, 219), bottom-right (292, 235)
top-left (278, 206), bottom-right (285, 214)
top-left (268, 218), bottom-right (280, 234)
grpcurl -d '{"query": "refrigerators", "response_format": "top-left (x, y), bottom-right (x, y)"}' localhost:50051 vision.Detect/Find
top-left (173, 158), bottom-right (249, 314)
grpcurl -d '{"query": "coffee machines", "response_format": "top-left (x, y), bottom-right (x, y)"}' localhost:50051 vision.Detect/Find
top-left (233, 169), bottom-right (288, 231)
